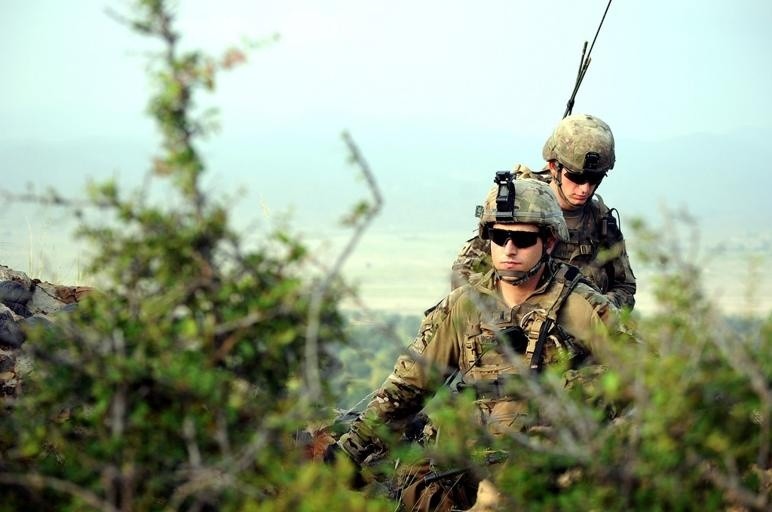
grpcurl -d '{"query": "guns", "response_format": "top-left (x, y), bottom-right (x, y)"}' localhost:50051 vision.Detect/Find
top-left (422, 451), bottom-right (507, 488)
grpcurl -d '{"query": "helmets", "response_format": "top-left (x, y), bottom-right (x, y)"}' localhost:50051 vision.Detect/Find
top-left (543, 113), bottom-right (615, 173)
top-left (475, 177), bottom-right (570, 243)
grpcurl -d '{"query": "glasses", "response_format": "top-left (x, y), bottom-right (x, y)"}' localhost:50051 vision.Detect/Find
top-left (554, 161), bottom-right (606, 184)
top-left (486, 227), bottom-right (544, 248)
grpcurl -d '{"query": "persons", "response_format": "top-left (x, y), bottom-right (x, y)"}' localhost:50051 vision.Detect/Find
top-left (451, 115), bottom-right (636, 317)
top-left (333, 171), bottom-right (635, 511)
